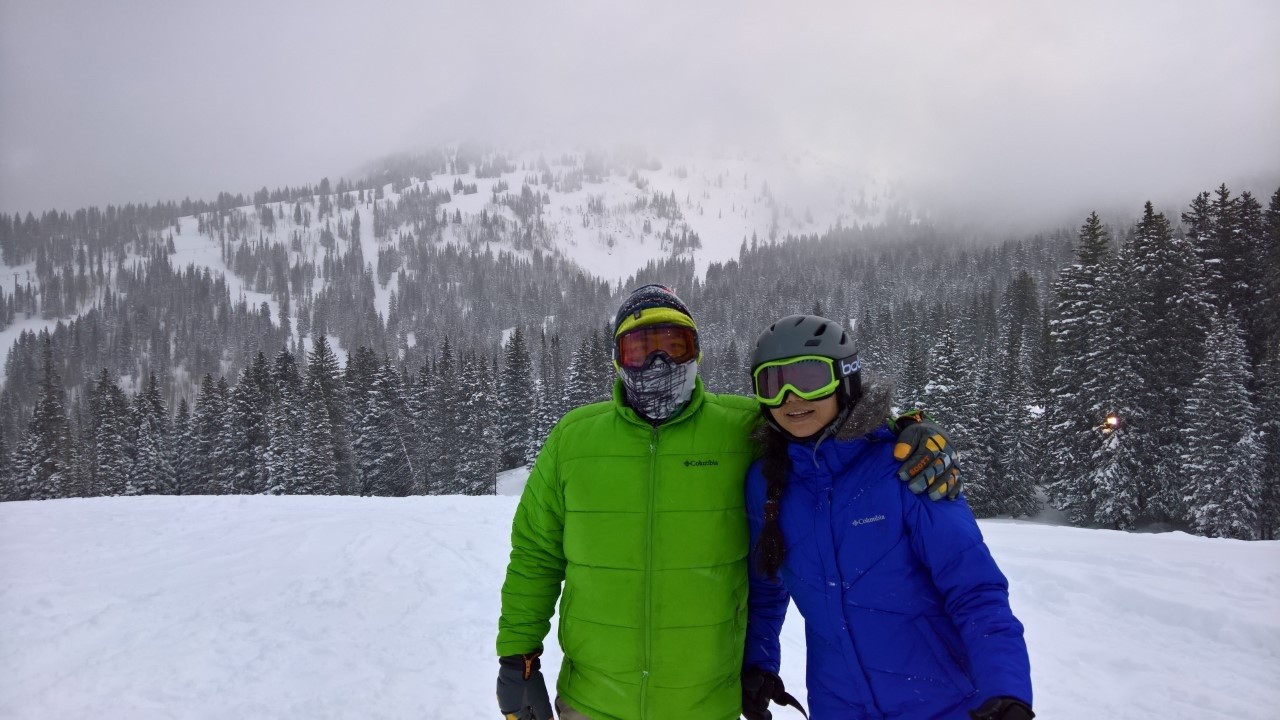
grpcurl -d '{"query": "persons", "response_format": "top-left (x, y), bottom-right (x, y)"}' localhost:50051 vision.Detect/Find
top-left (497, 285), bottom-right (963, 720)
top-left (740, 315), bottom-right (1033, 720)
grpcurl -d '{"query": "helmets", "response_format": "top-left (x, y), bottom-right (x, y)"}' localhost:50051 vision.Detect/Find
top-left (750, 314), bottom-right (865, 411)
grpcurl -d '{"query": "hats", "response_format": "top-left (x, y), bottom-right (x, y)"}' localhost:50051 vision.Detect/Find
top-left (611, 285), bottom-right (695, 339)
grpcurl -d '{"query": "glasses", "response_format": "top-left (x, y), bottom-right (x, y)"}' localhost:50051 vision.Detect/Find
top-left (754, 356), bottom-right (839, 407)
top-left (618, 327), bottom-right (696, 371)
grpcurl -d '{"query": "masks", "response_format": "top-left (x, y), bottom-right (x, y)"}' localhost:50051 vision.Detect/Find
top-left (618, 360), bottom-right (698, 420)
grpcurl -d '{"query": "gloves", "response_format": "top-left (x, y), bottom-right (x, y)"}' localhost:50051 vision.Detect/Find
top-left (496, 649), bottom-right (554, 720)
top-left (741, 665), bottom-right (788, 720)
top-left (970, 697), bottom-right (1036, 720)
top-left (893, 408), bottom-right (964, 500)
